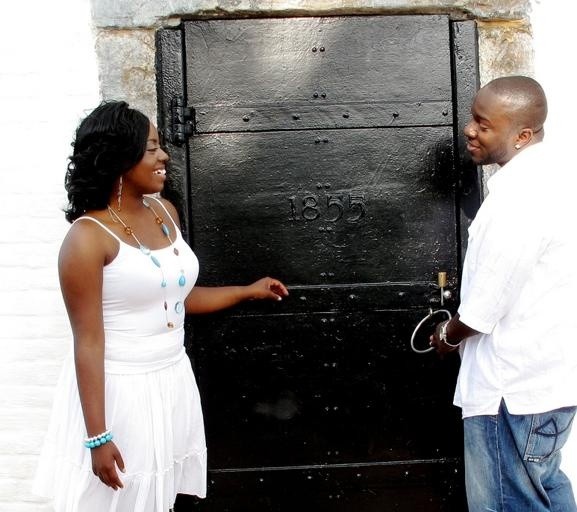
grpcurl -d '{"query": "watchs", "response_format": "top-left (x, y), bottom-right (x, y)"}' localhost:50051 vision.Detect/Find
top-left (439, 320), bottom-right (464, 350)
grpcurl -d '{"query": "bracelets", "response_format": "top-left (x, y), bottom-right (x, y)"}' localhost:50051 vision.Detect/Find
top-left (83, 430), bottom-right (112, 449)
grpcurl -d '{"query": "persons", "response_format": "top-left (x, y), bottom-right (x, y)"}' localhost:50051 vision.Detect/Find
top-left (429, 75), bottom-right (577, 512)
top-left (57, 100), bottom-right (289, 512)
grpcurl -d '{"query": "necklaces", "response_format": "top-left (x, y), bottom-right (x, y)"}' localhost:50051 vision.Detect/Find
top-left (106, 198), bottom-right (185, 329)
